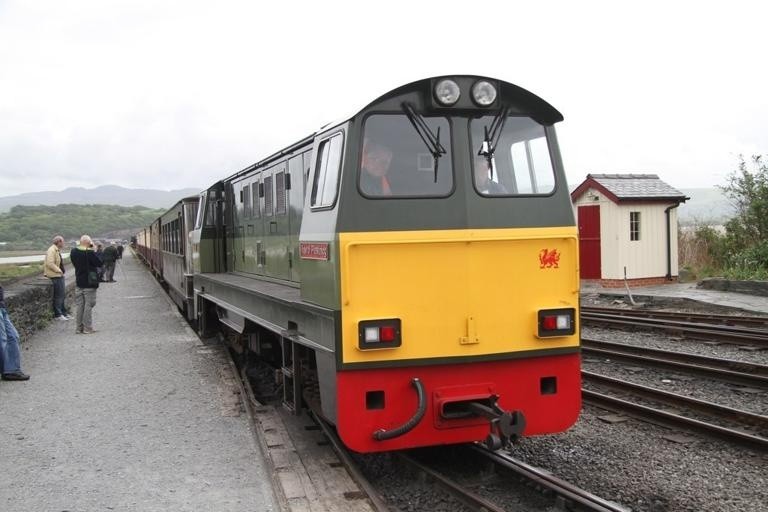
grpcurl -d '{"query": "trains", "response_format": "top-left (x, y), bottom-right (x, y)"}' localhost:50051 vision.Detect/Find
top-left (129, 71), bottom-right (586, 459)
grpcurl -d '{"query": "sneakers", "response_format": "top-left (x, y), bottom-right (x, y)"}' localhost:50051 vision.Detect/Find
top-left (76, 326), bottom-right (96, 334)
top-left (54, 314), bottom-right (74, 322)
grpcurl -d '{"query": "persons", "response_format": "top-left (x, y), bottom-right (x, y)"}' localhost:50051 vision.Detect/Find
top-left (474, 154), bottom-right (509, 195)
top-left (0, 285), bottom-right (30, 381)
top-left (44, 236), bottom-right (74, 321)
top-left (96, 241), bottom-right (124, 282)
top-left (70, 235), bottom-right (105, 334)
top-left (360, 139), bottom-right (427, 196)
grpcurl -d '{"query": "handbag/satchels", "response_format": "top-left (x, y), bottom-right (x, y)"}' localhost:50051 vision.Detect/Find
top-left (88, 271), bottom-right (97, 285)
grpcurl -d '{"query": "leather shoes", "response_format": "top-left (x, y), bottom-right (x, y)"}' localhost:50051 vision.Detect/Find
top-left (2, 370), bottom-right (30, 381)
